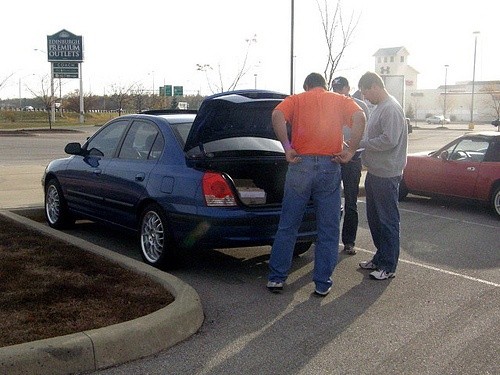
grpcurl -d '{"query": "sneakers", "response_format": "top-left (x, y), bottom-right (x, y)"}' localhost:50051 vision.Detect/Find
top-left (359, 261), bottom-right (378, 269)
top-left (344, 244), bottom-right (357, 255)
top-left (369, 267), bottom-right (396, 280)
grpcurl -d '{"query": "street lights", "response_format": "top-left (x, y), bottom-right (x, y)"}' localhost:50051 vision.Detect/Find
top-left (467, 31), bottom-right (480, 130)
top-left (442, 64), bottom-right (450, 124)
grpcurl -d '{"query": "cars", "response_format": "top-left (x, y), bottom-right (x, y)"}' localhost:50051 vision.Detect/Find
top-left (396, 131), bottom-right (500, 221)
top-left (41, 88), bottom-right (344, 274)
top-left (425, 115), bottom-right (451, 125)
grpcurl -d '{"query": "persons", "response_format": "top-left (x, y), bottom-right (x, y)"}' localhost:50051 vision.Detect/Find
top-left (330, 76), bottom-right (370, 255)
top-left (265, 71), bottom-right (367, 297)
top-left (347, 71), bottom-right (408, 282)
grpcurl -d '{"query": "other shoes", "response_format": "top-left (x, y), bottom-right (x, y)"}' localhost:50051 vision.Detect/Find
top-left (266, 281), bottom-right (283, 291)
top-left (314, 287), bottom-right (331, 296)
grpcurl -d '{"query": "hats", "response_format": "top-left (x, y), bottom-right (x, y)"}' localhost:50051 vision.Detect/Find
top-left (330, 77), bottom-right (348, 90)
